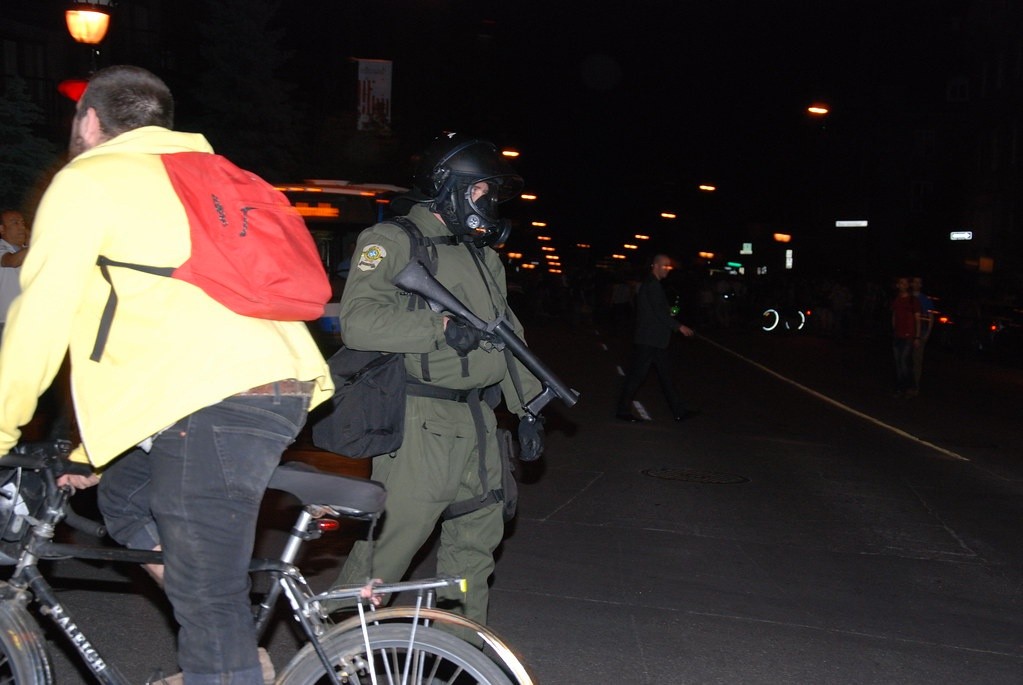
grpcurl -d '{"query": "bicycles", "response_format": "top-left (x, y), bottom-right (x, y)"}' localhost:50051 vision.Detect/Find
top-left (0, 437), bottom-right (537, 685)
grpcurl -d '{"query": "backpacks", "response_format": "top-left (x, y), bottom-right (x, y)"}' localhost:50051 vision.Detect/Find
top-left (96, 152), bottom-right (332, 321)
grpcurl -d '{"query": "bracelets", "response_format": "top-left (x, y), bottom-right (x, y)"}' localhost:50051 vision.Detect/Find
top-left (915, 337), bottom-right (921, 339)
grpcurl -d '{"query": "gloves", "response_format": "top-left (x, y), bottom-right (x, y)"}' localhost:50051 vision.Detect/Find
top-left (443, 315), bottom-right (503, 356)
top-left (517, 416), bottom-right (545, 461)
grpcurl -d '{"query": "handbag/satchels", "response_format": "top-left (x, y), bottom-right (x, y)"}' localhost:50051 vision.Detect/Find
top-left (311, 341), bottom-right (405, 459)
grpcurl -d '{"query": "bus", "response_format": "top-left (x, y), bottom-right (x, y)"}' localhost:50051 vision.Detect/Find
top-left (259, 172), bottom-right (419, 349)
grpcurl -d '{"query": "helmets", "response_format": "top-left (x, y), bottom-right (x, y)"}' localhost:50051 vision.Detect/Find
top-left (418, 138), bottom-right (525, 249)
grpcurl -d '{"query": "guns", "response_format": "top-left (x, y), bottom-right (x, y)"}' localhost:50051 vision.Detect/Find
top-left (390, 256), bottom-right (583, 419)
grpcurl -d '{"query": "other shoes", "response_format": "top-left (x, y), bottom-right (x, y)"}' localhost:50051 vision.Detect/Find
top-left (616, 407), bottom-right (644, 423)
top-left (672, 408), bottom-right (701, 423)
top-left (145, 647), bottom-right (276, 685)
top-left (905, 390), bottom-right (912, 399)
top-left (913, 386), bottom-right (919, 397)
top-left (893, 391), bottom-right (902, 398)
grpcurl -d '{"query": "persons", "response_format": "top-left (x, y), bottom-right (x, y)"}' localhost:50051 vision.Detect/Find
top-left (615, 254), bottom-right (706, 423)
top-left (891, 275), bottom-right (934, 399)
top-left (0, 64), bottom-right (334, 685)
top-left (286, 135), bottom-right (546, 685)
